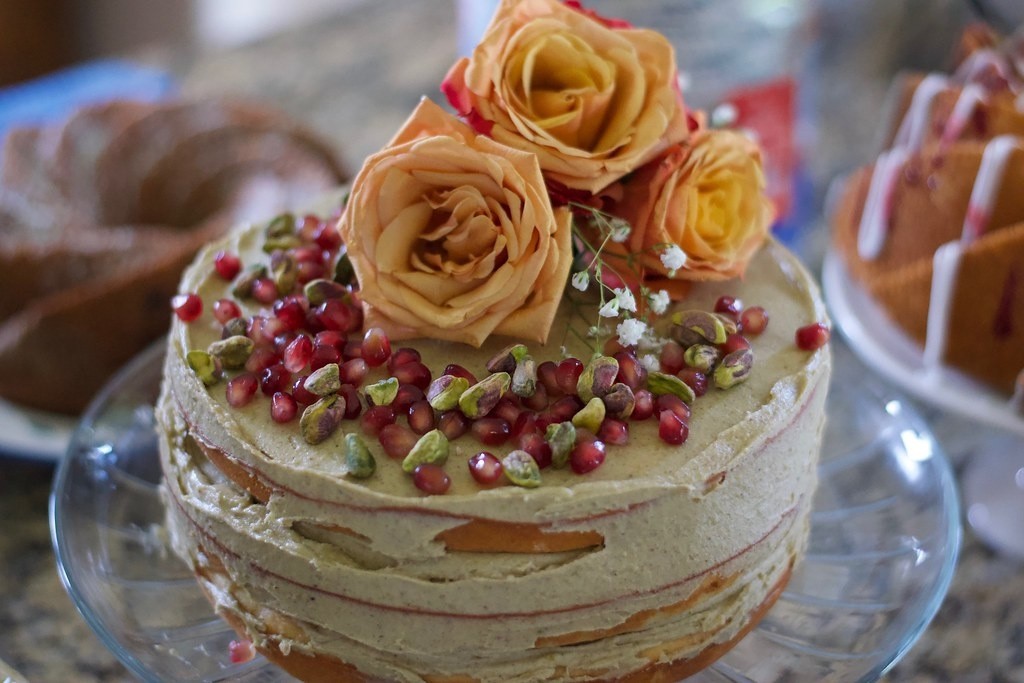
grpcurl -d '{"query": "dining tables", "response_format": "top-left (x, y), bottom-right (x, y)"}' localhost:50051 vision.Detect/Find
top-left (1, 6), bottom-right (1024, 679)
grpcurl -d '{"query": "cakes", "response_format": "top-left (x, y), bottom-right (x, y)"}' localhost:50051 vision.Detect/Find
top-left (156, 210), bottom-right (831, 682)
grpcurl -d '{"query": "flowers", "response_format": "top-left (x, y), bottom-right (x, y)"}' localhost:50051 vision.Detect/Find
top-left (336, 1), bottom-right (780, 352)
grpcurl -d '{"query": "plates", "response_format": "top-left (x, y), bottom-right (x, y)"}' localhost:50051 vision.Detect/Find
top-left (47, 328), bottom-right (962, 683)
top-left (0, 380), bottom-right (160, 471)
top-left (823, 244), bottom-right (1024, 432)
